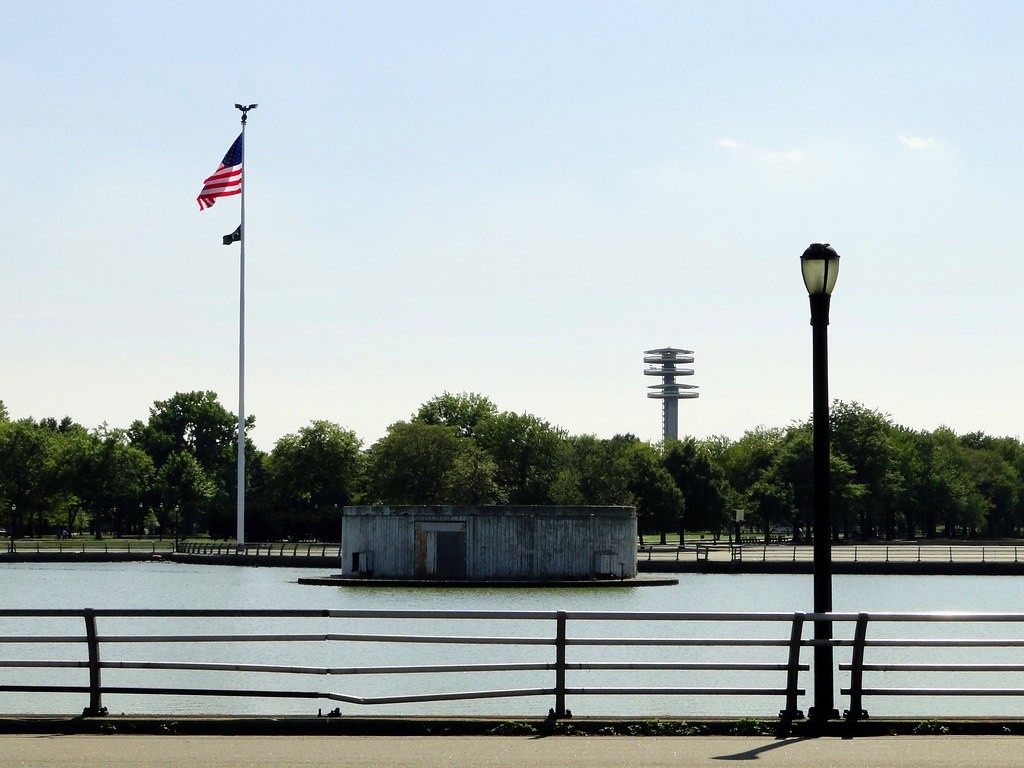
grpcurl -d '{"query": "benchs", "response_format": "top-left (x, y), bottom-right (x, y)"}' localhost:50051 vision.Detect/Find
top-left (736, 535), bottom-right (789, 543)
top-left (695, 543), bottom-right (742, 560)
top-left (240, 541), bottom-right (266, 554)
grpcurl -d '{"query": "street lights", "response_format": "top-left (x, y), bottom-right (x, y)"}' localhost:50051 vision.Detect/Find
top-left (799, 242), bottom-right (842, 719)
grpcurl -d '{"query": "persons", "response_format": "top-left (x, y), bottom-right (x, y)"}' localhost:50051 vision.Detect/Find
top-left (57, 528), bottom-right (67, 541)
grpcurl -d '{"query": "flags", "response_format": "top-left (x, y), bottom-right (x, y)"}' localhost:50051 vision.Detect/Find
top-left (196, 130), bottom-right (244, 211)
top-left (223, 223), bottom-right (242, 245)
